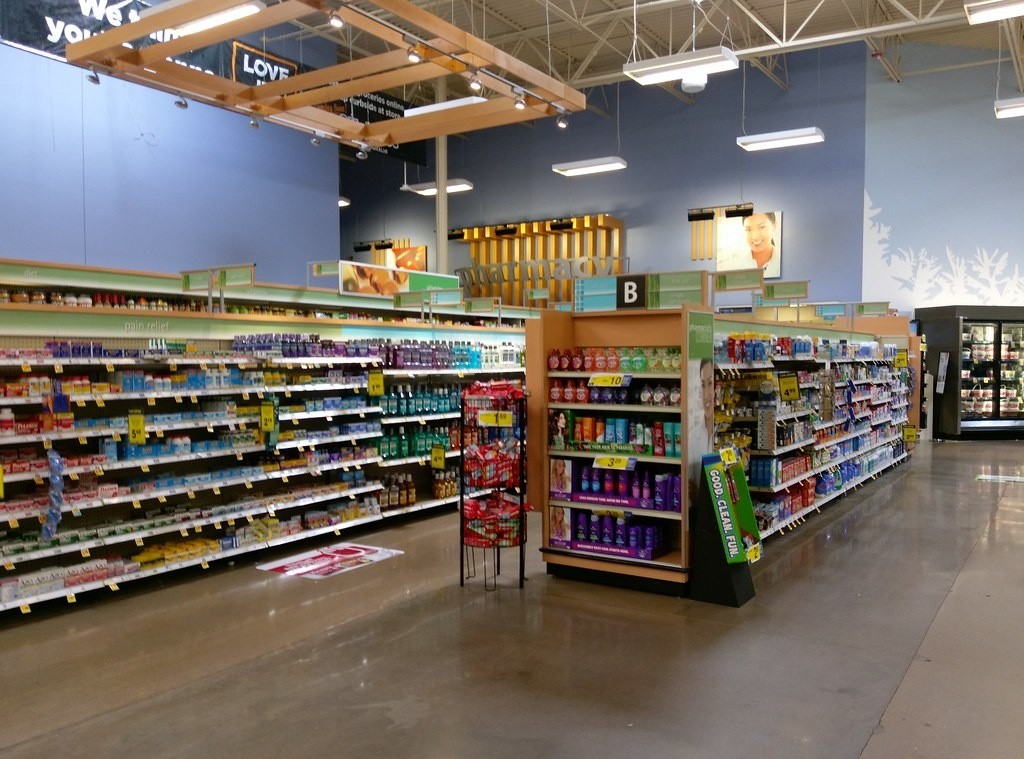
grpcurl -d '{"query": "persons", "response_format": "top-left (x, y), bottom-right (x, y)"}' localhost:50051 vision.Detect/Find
top-left (548, 411), bottom-right (568, 449)
top-left (551, 460), bottom-right (571, 492)
top-left (687, 358), bottom-right (713, 462)
top-left (717, 212), bottom-right (780, 277)
top-left (550, 507), bottom-right (570, 539)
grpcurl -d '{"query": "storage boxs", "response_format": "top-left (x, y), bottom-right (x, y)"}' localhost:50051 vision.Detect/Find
top-left (0, 337), bottom-right (380, 605)
top-left (550, 458), bottom-right (654, 509)
top-left (742, 339), bottom-right (910, 526)
top-left (547, 409), bottom-right (653, 454)
top-left (549, 506), bottom-right (665, 560)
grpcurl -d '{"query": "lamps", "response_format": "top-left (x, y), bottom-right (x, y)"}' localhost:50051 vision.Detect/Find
top-left (513, 93), bottom-right (525, 109)
top-left (995, 97), bottom-right (1024, 119)
top-left (495, 224), bottom-right (517, 236)
top-left (354, 242), bottom-right (371, 252)
top-left (248, 115), bottom-right (260, 128)
top-left (963, 0), bottom-right (1024, 26)
top-left (339, 196), bottom-right (351, 207)
top-left (549, 219), bottom-right (573, 231)
top-left (355, 147), bottom-right (368, 160)
top-left (375, 241), bottom-right (392, 249)
top-left (87, 71), bottom-right (100, 84)
top-left (141, 0), bottom-right (266, 37)
top-left (469, 72), bottom-right (482, 89)
top-left (310, 132), bottom-right (320, 146)
top-left (328, 9), bottom-right (344, 28)
top-left (406, 46), bottom-right (421, 64)
top-left (688, 211), bottom-right (715, 221)
top-left (734, 127), bottom-right (825, 151)
top-left (622, 45), bottom-right (740, 87)
top-left (448, 229), bottom-right (464, 240)
top-left (551, 156), bottom-right (628, 176)
top-left (404, 179), bottom-right (472, 197)
top-left (557, 114), bottom-right (568, 129)
top-left (725, 208), bottom-right (753, 218)
top-left (174, 95), bottom-right (188, 109)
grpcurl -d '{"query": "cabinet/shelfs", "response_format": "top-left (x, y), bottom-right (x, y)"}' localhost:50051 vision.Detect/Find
top-left (458, 393), bottom-right (527, 592)
top-left (915, 306), bottom-right (1024, 441)
top-left (524, 310), bottom-right (924, 598)
top-left (0, 260), bottom-right (527, 615)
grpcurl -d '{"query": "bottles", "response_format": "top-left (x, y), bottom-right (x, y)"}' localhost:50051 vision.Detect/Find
top-left (571, 417), bottom-right (680, 458)
top-left (582, 467), bottom-right (680, 511)
top-left (233, 333), bottom-right (322, 357)
top-left (379, 420), bottom-right (483, 461)
top-left (321, 338), bottom-right (379, 357)
top-left (379, 338), bottom-right (526, 370)
top-left (578, 514), bottom-right (654, 550)
top-left (549, 379), bottom-right (681, 406)
top-left (433, 466), bottom-right (460, 499)
top-left (379, 385), bottom-right (461, 418)
top-left (379, 473), bottom-right (416, 512)
top-left (548, 347), bottom-right (681, 373)
top-left (0, 285), bottom-right (287, 315)
